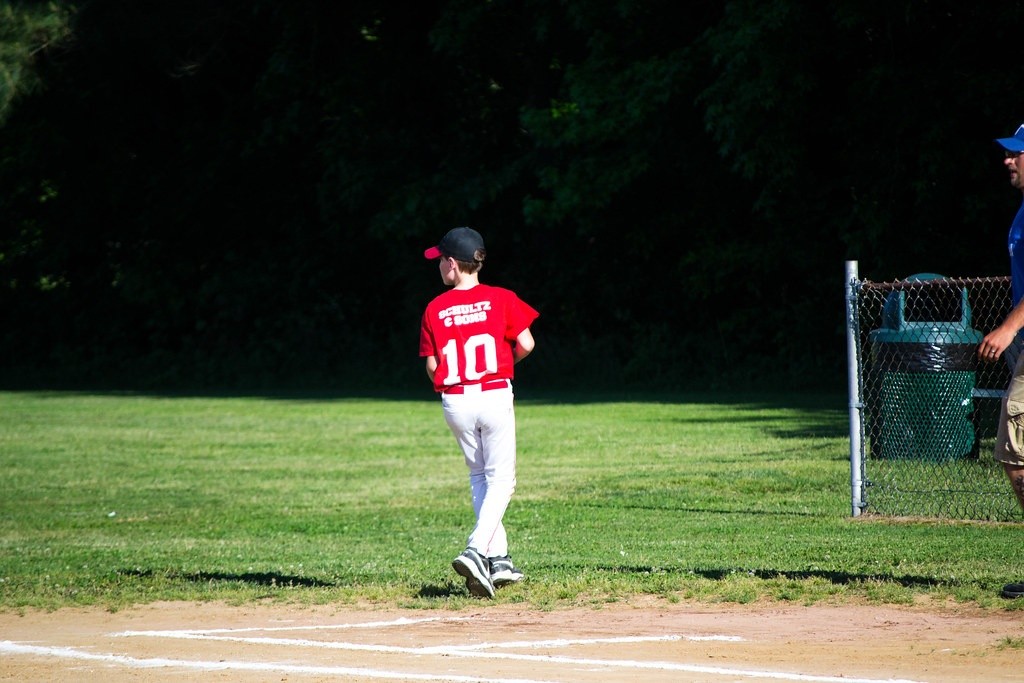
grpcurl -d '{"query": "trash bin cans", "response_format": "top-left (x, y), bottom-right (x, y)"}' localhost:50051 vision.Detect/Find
top-left (867, 272), bottom-right (986, 461)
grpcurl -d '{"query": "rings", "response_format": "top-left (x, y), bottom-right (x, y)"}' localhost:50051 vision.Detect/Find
top-left (990, 351), bottom-right (995, 353)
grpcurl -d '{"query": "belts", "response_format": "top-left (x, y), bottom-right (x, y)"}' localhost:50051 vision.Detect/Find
top-left (444, 381), bottom-right (508, 394)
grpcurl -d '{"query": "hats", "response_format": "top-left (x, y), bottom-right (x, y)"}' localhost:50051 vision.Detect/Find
top-left (993, 124), bottom-right (1024, 153)
top-left (424, 227), bottom-right (485, 263)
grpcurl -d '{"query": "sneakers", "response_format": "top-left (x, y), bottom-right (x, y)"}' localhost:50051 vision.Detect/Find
top-left (452, 546), bottom-right (495, 598)
top-left (488, 555), bottom-right (524, 588)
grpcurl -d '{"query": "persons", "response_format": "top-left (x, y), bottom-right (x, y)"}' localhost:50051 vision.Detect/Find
top-left (419, 226), bottom-right (540, 598)
top-left (977, 121), bottom-right (1024, 599)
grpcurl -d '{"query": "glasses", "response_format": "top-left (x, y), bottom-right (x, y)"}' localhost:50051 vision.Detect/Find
top-left (1004, 150), bottom-right (1024, 158)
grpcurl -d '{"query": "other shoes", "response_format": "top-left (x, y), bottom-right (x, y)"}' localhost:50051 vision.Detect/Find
top-left (1002, 584), bottom-right (1024, 599)
